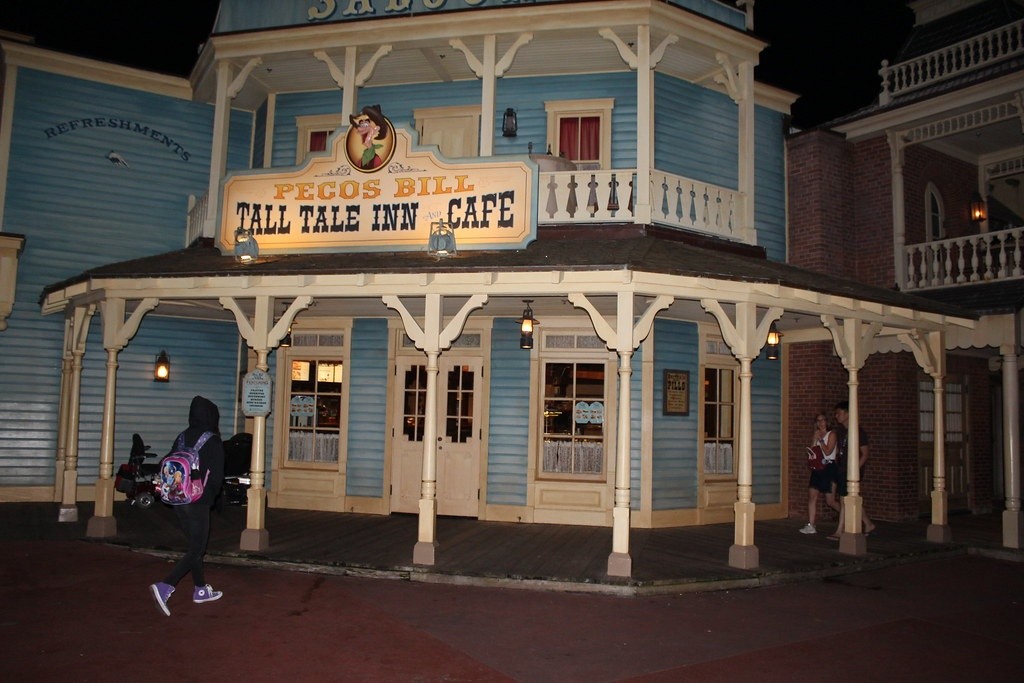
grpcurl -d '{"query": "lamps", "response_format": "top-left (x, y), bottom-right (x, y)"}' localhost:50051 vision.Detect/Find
top-left (232, 228), bottom-right (258, 263)
top-left (970, 193), bottom-right (987, 221)
top-left (767, 319), bottom-right (784, 360)
top-left (516, 299), bottom-right (541, 349)
top-left (152, 351), bottom-right (170, 383)
top-left (428, 219), bottom-right (458, 257)
top-left (277, 302), bottom-right (297, 347)
top-left (500, 107), bottom-right (518, 137)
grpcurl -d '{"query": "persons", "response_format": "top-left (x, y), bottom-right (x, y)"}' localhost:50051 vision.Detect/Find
top-left (147, 395), bottom-right (226, 617)
top-left (798, 401), bottom-right (877, 542)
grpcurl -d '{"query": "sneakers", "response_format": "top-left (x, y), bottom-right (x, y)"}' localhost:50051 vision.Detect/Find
top-left (193, 584), bottom-right (223, 604)
top-left (149, 582), bottom-right (175, 617)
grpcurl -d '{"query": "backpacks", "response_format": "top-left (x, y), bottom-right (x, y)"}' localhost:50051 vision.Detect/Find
top-left (159, 432), bottom-right (215, 505)
top-left (805, 445), bottom-right (837, 470)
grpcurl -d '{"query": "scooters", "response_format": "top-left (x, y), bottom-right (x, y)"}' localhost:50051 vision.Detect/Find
top-left (114, 432), bottom-right (254, 513)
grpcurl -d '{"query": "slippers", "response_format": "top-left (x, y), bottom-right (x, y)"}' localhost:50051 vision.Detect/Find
top-left (824, 535), bottom-right (841, 541)
top-left (863, 527), bottom-right (878, 538)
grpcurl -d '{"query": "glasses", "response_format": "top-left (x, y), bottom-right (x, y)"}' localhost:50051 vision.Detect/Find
top-left (816, 419), bottom-right (826, 423)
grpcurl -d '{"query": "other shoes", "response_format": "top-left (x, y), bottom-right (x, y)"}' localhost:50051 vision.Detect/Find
top-left (799, 523), bottom-right (817, 534)
